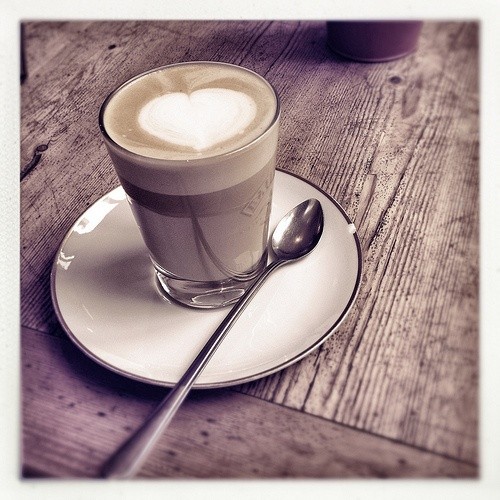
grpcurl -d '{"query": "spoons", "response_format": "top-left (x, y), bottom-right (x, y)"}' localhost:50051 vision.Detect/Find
top-left (90, 197), bottom-right (325, 480)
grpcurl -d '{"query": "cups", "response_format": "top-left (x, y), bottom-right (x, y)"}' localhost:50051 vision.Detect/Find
top-left (327, 20), bottom-right (425, 63)
top-left (97, 61), bottom-right (281, 310)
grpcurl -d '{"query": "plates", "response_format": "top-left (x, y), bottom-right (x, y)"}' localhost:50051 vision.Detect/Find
top-left (50, 168), bottom-right (363, 389)
